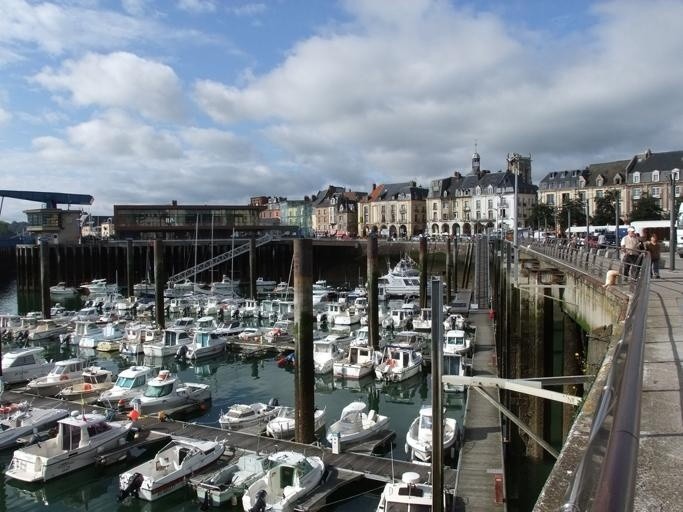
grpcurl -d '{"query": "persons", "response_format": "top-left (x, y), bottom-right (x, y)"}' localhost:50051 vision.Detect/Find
top-left (555, 229), bottom-right (607, 248)
top-left (619, 226), bottom-right (661, 281)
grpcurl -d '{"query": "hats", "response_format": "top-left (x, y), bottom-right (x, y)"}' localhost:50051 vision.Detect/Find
top-left (627, 226), bottom-right (635, 232)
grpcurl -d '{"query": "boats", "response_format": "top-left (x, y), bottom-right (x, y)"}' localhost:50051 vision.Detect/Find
top-left (5, 395), bottom-right (135, 483)
top-left (218, 398), bottom-right (279, 436)
top-left (1, 344), bottom-right (54, 385)
top-left (241, 454), bottom-right (326, 512)
top-left (116, 439), bottom-right (226, 503)
top-left (325, 400), bottom-right (390, 445)
top-left (96, 365), bottom-right (156, 409)
top-left (191, 450), bottom-right (305, 507)
top-left (27, 357), bottom-right (104, 395)
top-left (403, 404), bottom-right (459, 466)
top-left (374, 470), bottom-right (451, 512)
top-left (265, 406), bottom-right (327, 441)
top-left (128, 368), bottom-right (213, 420)
top-left (1, 401), bottom-right (70, 450)
top-left (56, 367), bottom-right (116, 405)
